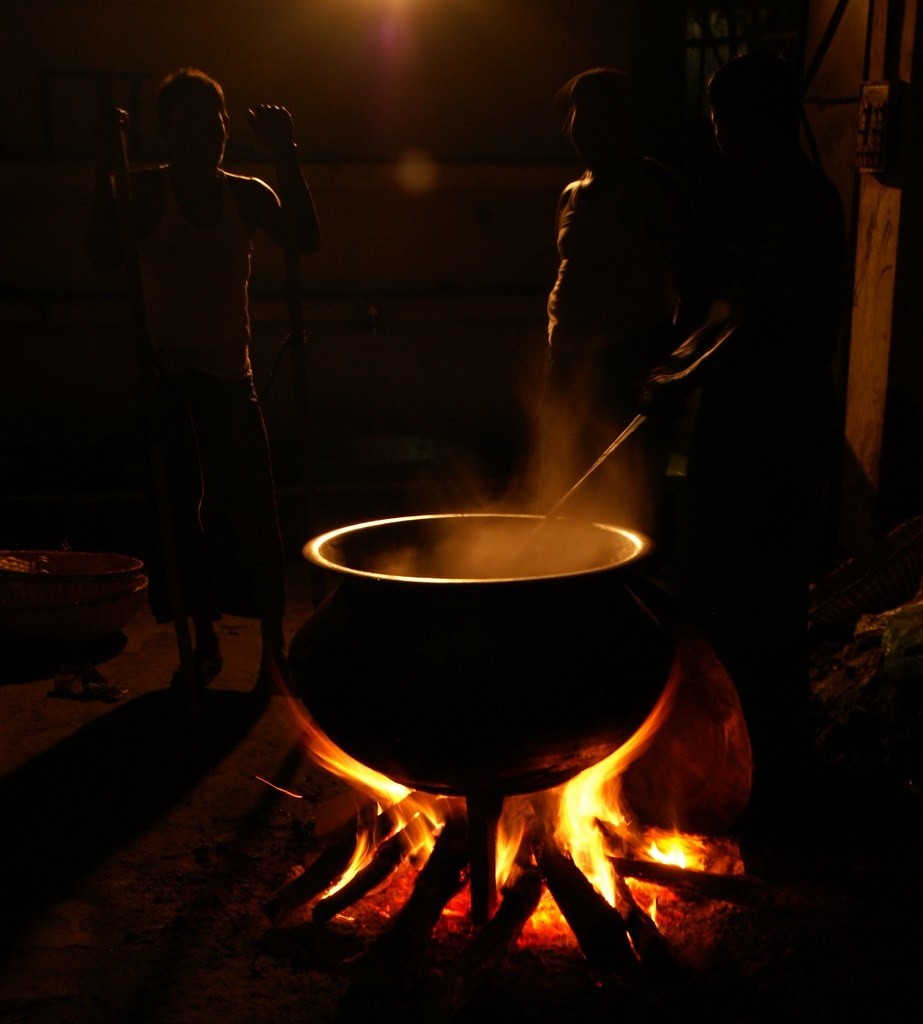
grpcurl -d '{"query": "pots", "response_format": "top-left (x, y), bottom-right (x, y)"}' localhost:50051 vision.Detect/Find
top-left (302, 512), bottom-right (655, 598)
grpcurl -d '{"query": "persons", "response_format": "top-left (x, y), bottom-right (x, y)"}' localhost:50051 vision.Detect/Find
top-left (534, 53), bottom-right (855, 809)
top-left (83, 65), bottom-right (321, 693)
